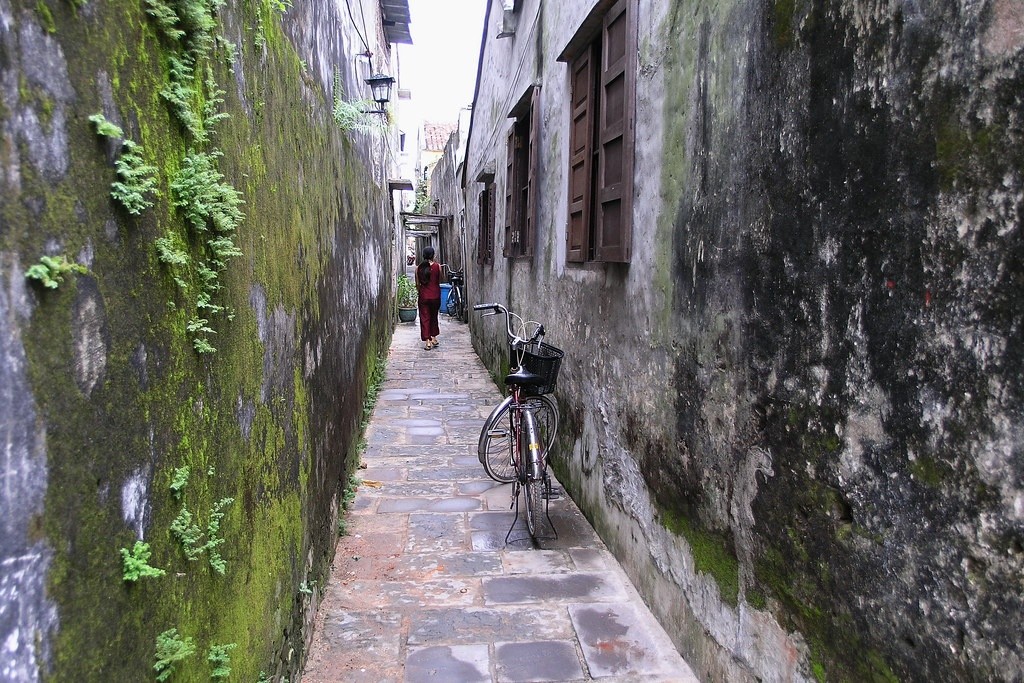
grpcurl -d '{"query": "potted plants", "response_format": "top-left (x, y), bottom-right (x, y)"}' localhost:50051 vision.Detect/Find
top-left (398, 273), bottom-right (417, 320)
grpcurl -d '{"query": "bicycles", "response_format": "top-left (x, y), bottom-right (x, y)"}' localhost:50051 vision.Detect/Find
top-left (473, 303), bottom-right (565, 544)
top-left (441, 264), bottom-right (464, 321)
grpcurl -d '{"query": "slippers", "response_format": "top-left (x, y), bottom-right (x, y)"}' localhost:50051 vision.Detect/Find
top-left (424, 346), bottom-right (431, 350)
top-left (433, 342), bottom-right (440, 346)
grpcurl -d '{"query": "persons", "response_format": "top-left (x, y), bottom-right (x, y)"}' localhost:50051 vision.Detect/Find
top-left (415, 246), bottom-right (442, 350)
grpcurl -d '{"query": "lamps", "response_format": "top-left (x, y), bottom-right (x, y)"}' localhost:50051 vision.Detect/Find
top-left (358, 74), bottom-right (396, 113)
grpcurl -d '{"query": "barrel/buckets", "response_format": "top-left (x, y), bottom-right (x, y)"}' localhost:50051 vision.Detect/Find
top-left (439, 284), bottom-right (453, 313)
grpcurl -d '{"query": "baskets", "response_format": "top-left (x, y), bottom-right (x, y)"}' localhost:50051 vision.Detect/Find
top-left (448, 270), bottom-right (464, 285)
top-left (509, 338), bottom-right (564, 394)
